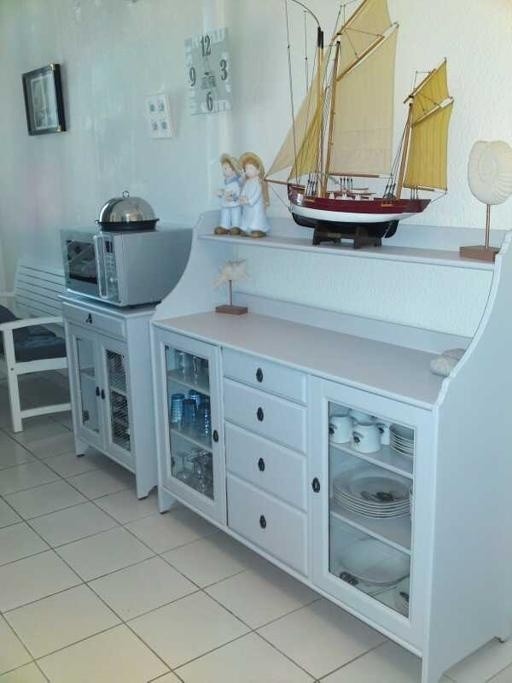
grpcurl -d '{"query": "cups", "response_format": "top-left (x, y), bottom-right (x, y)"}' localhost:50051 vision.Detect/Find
top-left (329, 409), bottom-right (390, 454)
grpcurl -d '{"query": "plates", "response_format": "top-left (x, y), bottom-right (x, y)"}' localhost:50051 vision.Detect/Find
top-left (96, 218), bottom-right (159, 231)
top-left (333, 462), bottom-right (413, 521)
top-left (391, 424), bottom-right (413, 461)
top-left (340, 537), bottom-right (410, 615)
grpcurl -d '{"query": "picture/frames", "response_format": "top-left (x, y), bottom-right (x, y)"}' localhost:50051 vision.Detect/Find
top-left (21, 62), bottom-right (68, 136)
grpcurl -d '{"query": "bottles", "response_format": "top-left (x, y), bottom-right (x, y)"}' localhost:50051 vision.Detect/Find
top-left (169, 351), bottom-right (207, 386)
top-left (171, 390), bottom-right (210, 443)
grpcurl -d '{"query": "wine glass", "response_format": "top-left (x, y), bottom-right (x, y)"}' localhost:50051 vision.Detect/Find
top-left (174, 452), bottom-right (192, 481)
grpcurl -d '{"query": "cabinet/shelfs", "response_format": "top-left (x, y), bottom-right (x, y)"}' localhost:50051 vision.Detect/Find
top-left (145, 206), bottom-right (512, 675)
top-left (55, 293), bottom-right (162, 499)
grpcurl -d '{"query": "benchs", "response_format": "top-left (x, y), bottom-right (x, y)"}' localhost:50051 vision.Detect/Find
top-left (0, 257), bottom-right (65, 433)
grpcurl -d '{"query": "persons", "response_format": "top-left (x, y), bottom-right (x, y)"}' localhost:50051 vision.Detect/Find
top-left (212, 152), bottom-right (245, 237)
top-left (233, 151), bottom-right (272, 239)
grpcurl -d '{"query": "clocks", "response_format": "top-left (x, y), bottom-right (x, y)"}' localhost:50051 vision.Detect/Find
top-left (182, 26), bottom-right (237, 116)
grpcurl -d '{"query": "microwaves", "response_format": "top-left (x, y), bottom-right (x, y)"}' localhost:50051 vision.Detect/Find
top-left (57, 226), bottom-right (192, 307)
